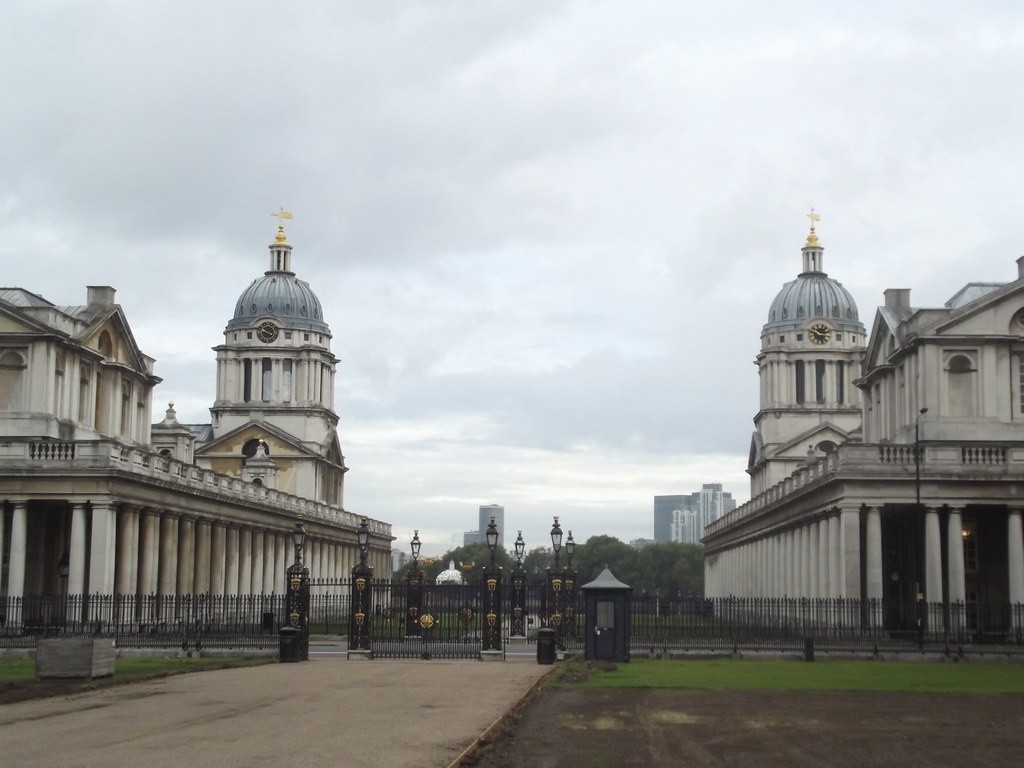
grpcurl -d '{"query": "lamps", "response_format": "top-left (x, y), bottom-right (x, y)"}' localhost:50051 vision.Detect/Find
top-left (292, 514), bottom-right (306, 559)
top-left (514, 530), bottom-right (526, 565)
top-left (486, 516), bottom-right (499, 561)
top-left (550, 516), bottom-right (563, 559)
top-left (410, 530), bottom-right (422, 565)
top-left (565, 530), bottom-right (575, 563)
top-left (357, 518), bottom-right (371, 560)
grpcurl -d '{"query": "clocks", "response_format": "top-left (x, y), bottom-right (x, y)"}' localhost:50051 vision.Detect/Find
top-left (808, 323), bottom-right (831, 345)
top-left (256, 321), bottom-right (279, 343)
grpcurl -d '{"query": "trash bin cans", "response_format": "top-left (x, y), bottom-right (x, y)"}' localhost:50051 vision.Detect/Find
top-left (536, 628), bottom-right (556, 664)
top-left (279, 626), bottom-right (302, 662)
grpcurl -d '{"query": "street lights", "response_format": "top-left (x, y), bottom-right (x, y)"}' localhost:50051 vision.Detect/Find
top-left (915, 407), bottom-right (929, 650)
